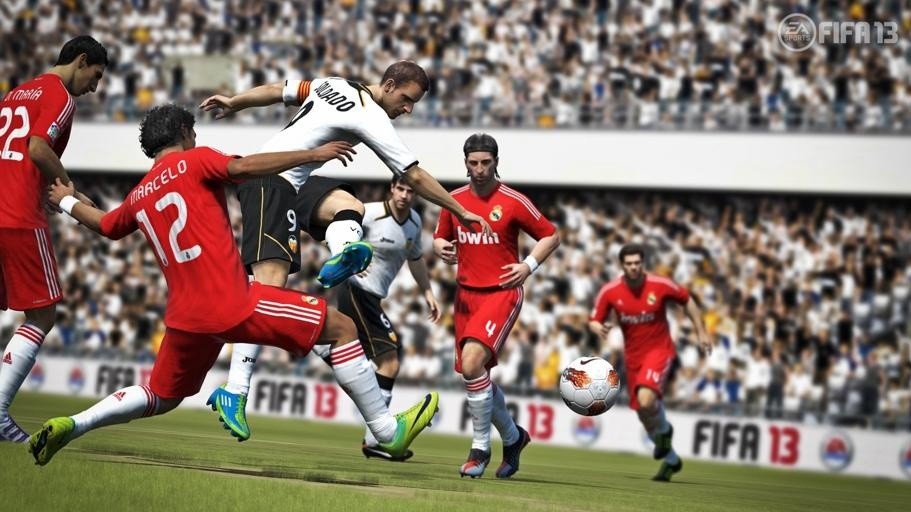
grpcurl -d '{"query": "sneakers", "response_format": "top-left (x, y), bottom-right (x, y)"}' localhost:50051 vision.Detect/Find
top-left (495, 426), bottom-right (531, 478)
top-left (457, 444), bottom-right (493, 479)
top-left (651, 428), bottom-right (685, 486)
top-left (360, 391), bottom-right (440, 463)
top-left (25, 415), bottom-right (75, 469)
top-left (0, 398), bottom-right (33, 454)
top-left (316, 239), bottom-right (370, 291)
top-left (206, 384), bottom-right (254, 445)
